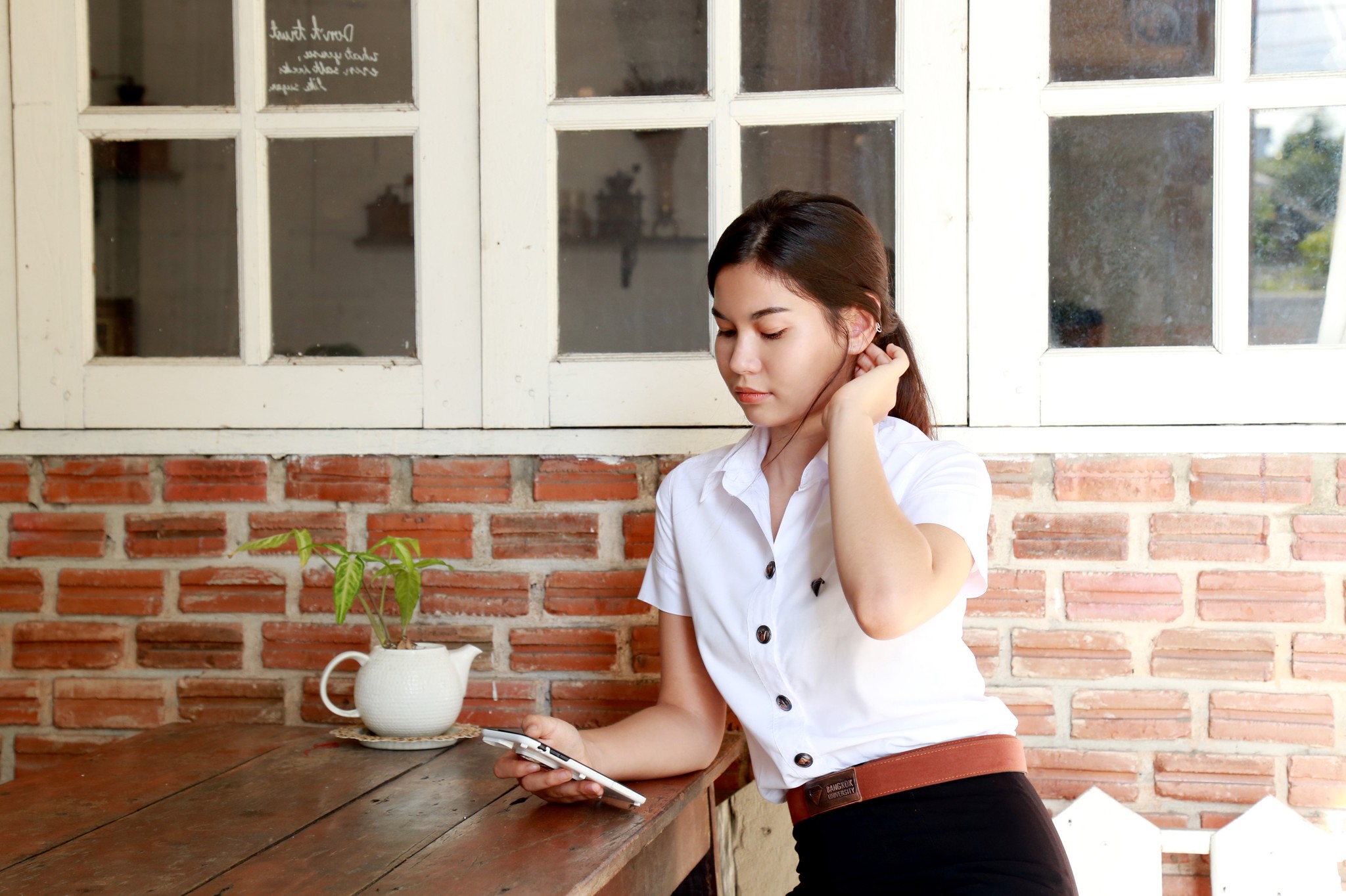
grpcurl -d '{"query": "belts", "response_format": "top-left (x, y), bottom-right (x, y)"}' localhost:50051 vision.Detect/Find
top-left (786, 735), bottom-right (1028, 826)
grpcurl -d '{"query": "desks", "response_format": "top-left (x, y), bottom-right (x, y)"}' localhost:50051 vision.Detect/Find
top-left (0, 724), bottom-right (753, 896)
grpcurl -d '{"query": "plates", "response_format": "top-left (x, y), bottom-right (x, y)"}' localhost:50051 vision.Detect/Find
top-left (329, 722), bottom-right (483, 751)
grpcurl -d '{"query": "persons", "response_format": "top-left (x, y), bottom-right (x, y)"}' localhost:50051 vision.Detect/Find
top-left (492, 188), bottom-right (1080, 896)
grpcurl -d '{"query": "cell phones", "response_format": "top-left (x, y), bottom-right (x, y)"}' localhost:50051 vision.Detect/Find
top-left (482, 728), bottom-right (646, 807)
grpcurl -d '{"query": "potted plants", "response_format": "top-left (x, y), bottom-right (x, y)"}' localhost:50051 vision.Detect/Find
top-left (234, 528), bottom-right (484, 751)
